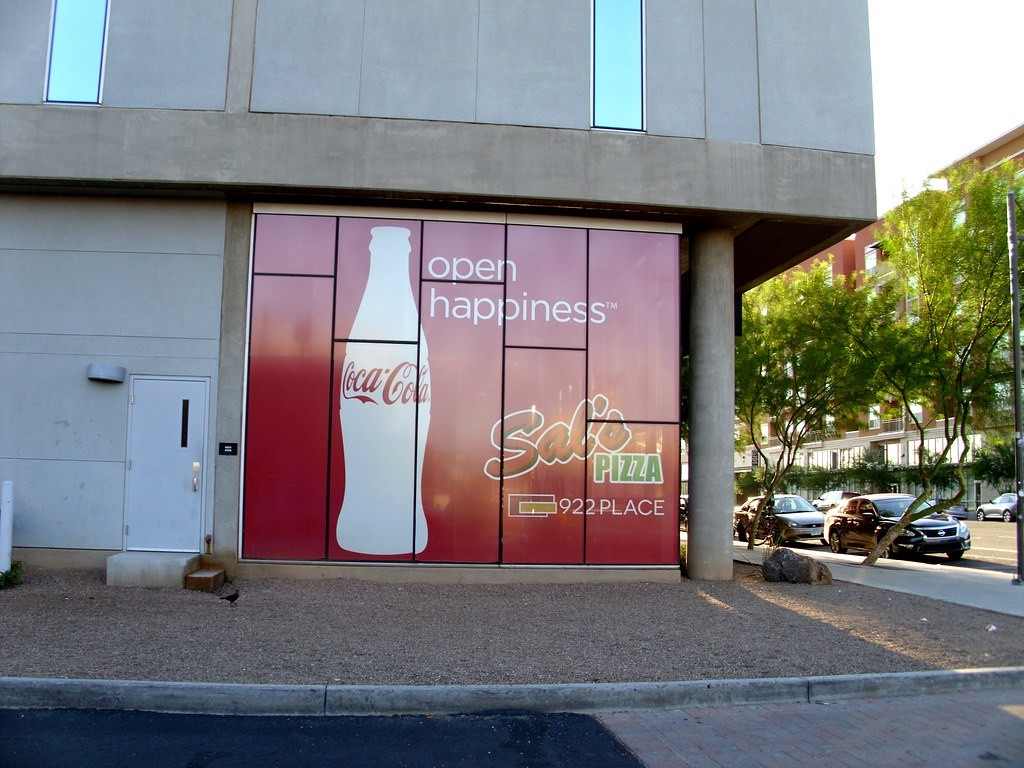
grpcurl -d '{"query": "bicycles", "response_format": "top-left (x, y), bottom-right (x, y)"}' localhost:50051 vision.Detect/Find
top-left (744, 499), bottom-right (791, 547)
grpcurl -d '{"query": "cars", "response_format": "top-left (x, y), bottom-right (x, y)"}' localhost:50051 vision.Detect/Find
top-left (976, 492), bottom-right (1018, 522)
top-left (734, 494), bottom-right (826, 548)
top-left (929, 498), bottom-right (968, 520)
top-left (680, 495), bottom-right (688, 514)
top-left (823, 493), bottom-right (971, 559)
top-left (812, 491), bottom-right (861, 511)
